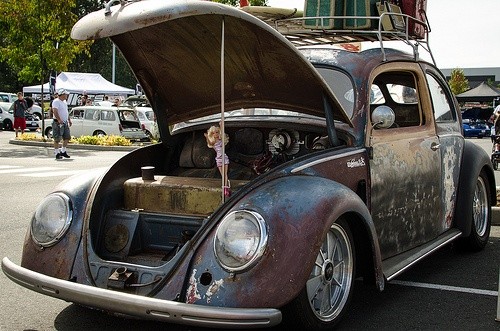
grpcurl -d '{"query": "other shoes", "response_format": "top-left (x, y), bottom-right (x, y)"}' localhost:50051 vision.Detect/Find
top-left (60, 151), bottom-right (70, 158)
top-left (56, 153), bottom-right (63, 159)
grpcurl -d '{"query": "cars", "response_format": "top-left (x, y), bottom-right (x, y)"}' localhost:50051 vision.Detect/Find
top-left (0, 106), bottom-right (20, 130)
top-left (0, 0), bottom-right (497, 331)
top-left (77, 94), bottom-right (152, 108)
top-left (0, 92), bottom-right (42, 131)
top-left (462, 123), bottom-right (491, 139)
top-left (131, 106), bottom-right (160, 142)
top-left (36, 105), bottom-right (149, 143)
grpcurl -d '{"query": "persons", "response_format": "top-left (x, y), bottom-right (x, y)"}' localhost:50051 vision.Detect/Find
top-left (99, 96), bottom-right (121, 106)
top-left (490, 105), bottom-right (500, 152)
top-left (52, 89), bottom-right (72, 160)
top-left (76, 94), bottom-right (92, 106)
top-left (14, 92), bottom-right (28, 137)
top-left (204, 121), bottom-right (231, 197)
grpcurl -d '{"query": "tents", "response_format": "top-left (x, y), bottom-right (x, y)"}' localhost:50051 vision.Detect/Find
top-left (455, 81), bottom-right (500, 107)
top-left (23, 72), bottom-right (136, 104)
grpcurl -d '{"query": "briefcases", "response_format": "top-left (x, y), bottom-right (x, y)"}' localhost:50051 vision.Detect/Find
top-left (376, 1), bottom-right (406, 32)
top-left (399, 0), bottom-right (431, 38)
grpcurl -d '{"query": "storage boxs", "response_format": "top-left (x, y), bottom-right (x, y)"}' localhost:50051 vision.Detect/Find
top-left (300, 0), bottom-right (433, 40)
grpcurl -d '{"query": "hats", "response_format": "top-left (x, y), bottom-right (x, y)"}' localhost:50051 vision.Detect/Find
top-left (58, 89), bottom-right (70, 95)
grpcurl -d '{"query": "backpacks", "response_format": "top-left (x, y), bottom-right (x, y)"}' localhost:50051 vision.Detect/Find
top-left (23, 98), bottom-right (34, 108)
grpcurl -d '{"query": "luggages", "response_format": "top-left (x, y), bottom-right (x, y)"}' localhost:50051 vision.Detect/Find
top-left (344, 0), bottom-right (380, 30)
top-left (303, 0), bottom-right (344, 29)
